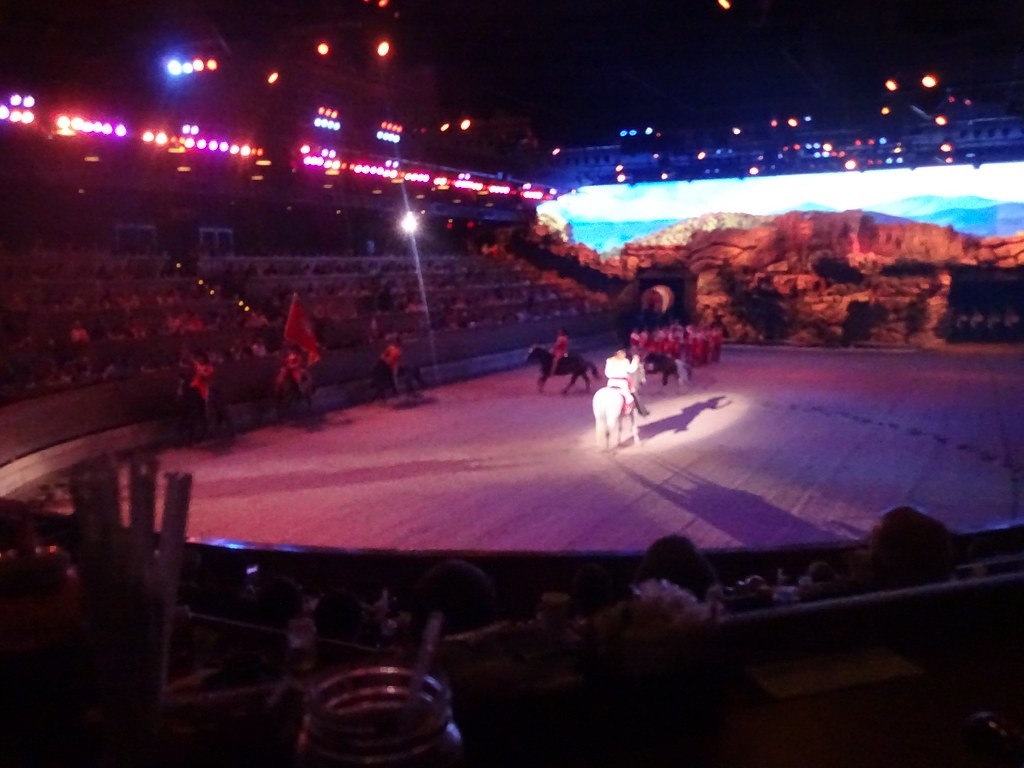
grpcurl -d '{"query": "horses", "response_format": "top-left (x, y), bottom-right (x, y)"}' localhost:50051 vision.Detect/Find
top-left (172, 358), bottom-right (428, 450)
top-left (592, 362), bottom-right (648, 451)
top-left (525, 344), bottom-right (600, 395)
top-left (643, 352), bottom-right (693, 392)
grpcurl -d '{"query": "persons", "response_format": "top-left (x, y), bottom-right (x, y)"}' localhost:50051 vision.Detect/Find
top-left (182, 349), bottom-right (215, 408)
top-left (1, 503), bottom-right (955, 768)
top-left (377, 337), bottom-right (406, 394)
top-left (604, 349), bottom-right (641, 417)
top-left (279, 344), bottom-right (307, 391)
top-left (549, 327), bottom-right (568, 379)
top-left (627, 320), bottom-right (726, 367)
